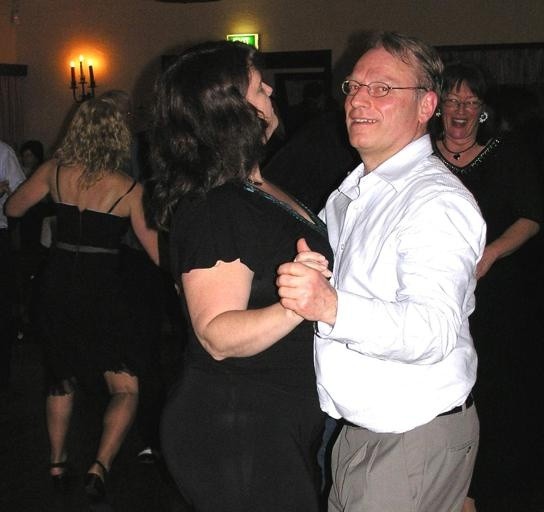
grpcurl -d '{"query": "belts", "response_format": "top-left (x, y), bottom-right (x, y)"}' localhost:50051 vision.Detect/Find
top-left (437, 394), bottom-right (473, 416)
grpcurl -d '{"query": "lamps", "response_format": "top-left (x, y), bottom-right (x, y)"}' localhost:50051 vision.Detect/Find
top-left (67, 55), bottom-right (98, 103)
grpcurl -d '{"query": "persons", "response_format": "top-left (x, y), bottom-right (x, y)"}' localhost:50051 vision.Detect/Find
top-left (20, 140), bottom-right (44, 178)
top-left (274, 30), bottom-right (487, 510)
top-left (132, 41), bottom-right (334, 509)
top-left (3, 99), bottom-right (159, 497)
top-left (0, 140), bottom-right (26, 339)
top-left (100, 90), bottom-right (133, 130)
top-left (286, 82), bottom-right (328, 112)
top-left (429, 66), bottom-right (542, 510)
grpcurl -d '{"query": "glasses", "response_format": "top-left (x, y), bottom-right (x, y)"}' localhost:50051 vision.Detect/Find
top-left (341, 80), bottom-right (428, 97)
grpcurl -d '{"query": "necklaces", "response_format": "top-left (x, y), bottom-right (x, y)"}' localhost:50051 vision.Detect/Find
top-left (244, 178), bottom-right (267, 187)
top-left (440, 138), bottom-right (478, 159)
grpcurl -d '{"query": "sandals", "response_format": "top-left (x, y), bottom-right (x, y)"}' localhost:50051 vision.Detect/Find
top-left (50, 461), bottom-right (70, 488)
top-left (84, 460), bottom-right (109, 500)
